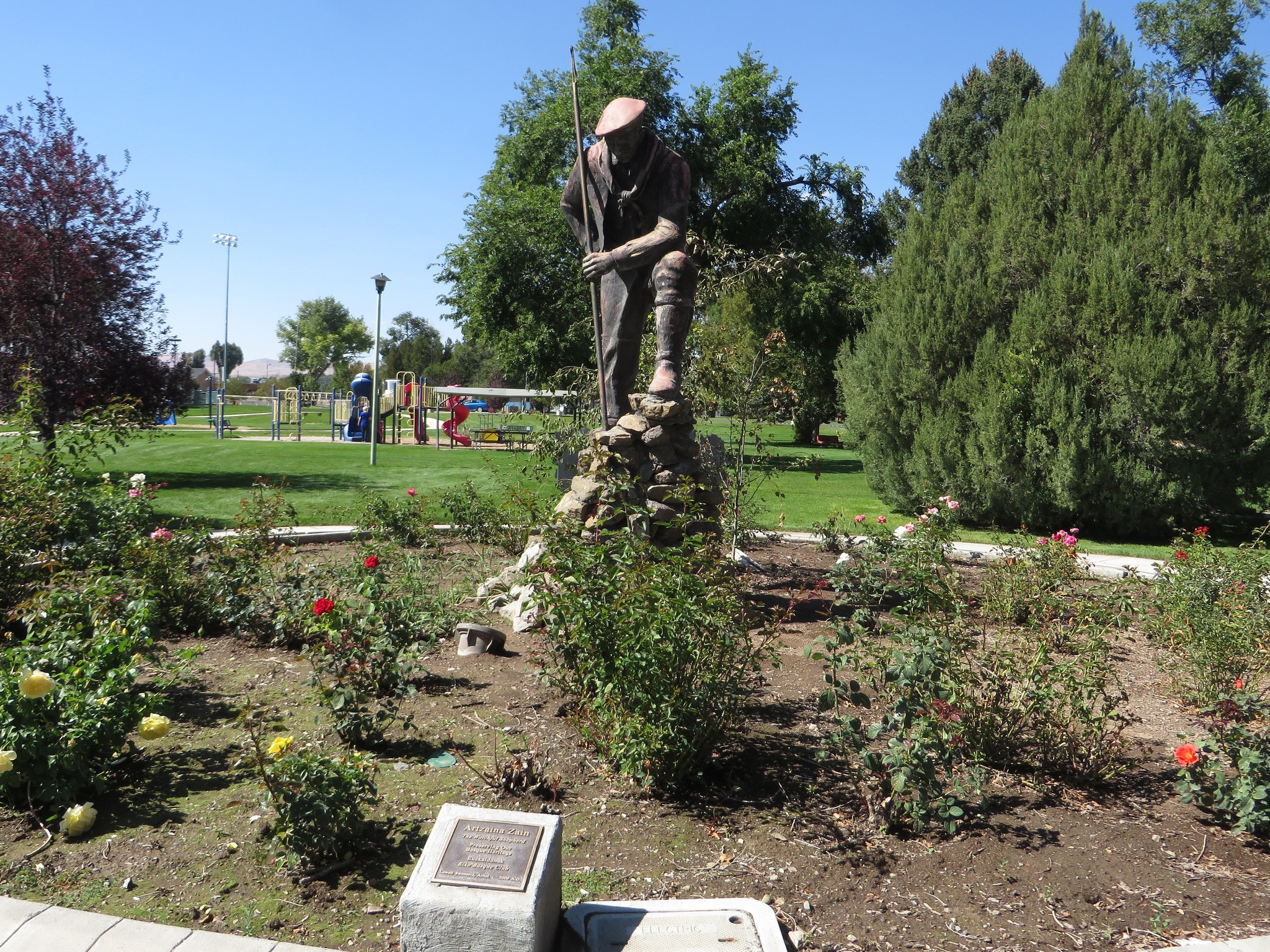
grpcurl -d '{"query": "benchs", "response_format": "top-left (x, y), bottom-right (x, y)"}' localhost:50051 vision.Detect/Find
top-left (467, 439), bottom-right (536, 452)
top-left (213, 420), bottom-right (239, 435)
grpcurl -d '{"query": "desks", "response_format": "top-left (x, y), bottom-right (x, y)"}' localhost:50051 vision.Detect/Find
top-left (468, 430), bottom-right (555, 450)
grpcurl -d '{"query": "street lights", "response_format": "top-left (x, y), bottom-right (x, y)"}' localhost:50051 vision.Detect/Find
top-left (213, 233), bottom-right (240, 439)
top-left (204, 375), bottom-right (212, 428)
top-left (368, 272), bottom-right (392, 467)
top-left (429, 344), bottom-right (433, 387)
top-left (267, 363), bottom-right (271, 381)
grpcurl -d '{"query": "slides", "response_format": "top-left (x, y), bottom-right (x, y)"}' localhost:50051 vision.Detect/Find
top-left (409, 409), bottom-right (430, 442)
top-left (441, 399), bottom-right (472, 446)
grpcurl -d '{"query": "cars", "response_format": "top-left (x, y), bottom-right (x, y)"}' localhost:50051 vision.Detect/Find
top-left (462, 398), bottom-right (489, 413)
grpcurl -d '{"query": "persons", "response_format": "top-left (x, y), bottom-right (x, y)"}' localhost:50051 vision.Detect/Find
top-left (351, 401), bottom-right (371, 442)
top-left (560, 98), bottom-right (699, 428)
